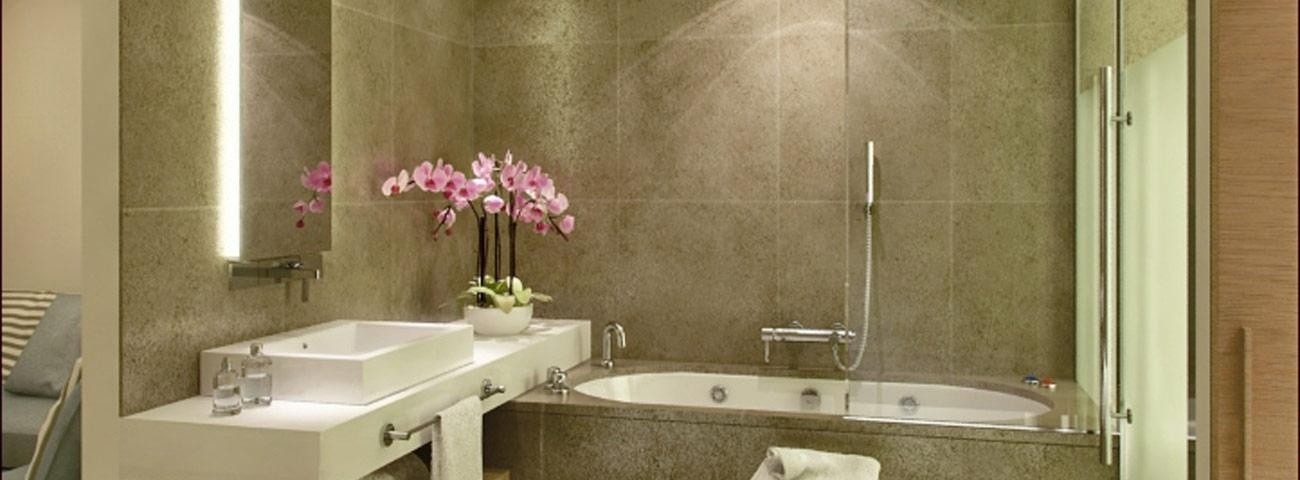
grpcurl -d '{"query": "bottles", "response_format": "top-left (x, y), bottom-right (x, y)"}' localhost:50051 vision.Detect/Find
top-left (241, 341), bottom-right (272, 406)
top-left (212, 359), bottom-right (241, 416)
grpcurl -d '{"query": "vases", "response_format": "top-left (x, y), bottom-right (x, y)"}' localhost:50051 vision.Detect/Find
top-left (462, 304), bottom-right (534, 337)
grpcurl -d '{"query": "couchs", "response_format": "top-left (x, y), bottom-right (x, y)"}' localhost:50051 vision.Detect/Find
top-left (2, 289), bottom-right (81, 479)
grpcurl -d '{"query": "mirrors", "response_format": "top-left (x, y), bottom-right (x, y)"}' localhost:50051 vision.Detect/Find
top-left (240, 0), bottom-right (331, 259)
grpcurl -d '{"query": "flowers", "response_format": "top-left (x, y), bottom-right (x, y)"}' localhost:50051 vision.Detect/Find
top-left (295, 160), bottom-right (330, 226)
top-left (381, 147), bottom-right (574, 312)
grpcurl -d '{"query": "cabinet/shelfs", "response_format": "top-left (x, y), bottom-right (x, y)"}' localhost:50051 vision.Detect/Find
top-left (1210, 1), bottom-right (1299, 480)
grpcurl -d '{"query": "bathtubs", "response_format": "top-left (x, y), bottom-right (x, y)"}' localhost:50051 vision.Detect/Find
top-left (484, 354), bottom-right (1119, 479)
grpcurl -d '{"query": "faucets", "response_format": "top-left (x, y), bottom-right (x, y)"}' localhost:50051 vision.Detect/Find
top-left (600, 320), bottom-right (628, 369)
top-left (255, 254), bottom-right (320, 303)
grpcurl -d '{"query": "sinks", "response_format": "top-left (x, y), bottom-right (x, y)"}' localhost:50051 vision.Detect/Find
top-left (200, 320), bottom-right (473, 404)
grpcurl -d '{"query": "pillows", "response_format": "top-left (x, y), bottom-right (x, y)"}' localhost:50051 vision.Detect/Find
top-left (4, 294), bottom-right (81, 396)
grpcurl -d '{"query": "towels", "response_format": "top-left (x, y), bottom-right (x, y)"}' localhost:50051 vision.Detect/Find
top-left (431, 396), bottom-right (484, 480)
top-left (749, 446), bottom-right (881, 480)
